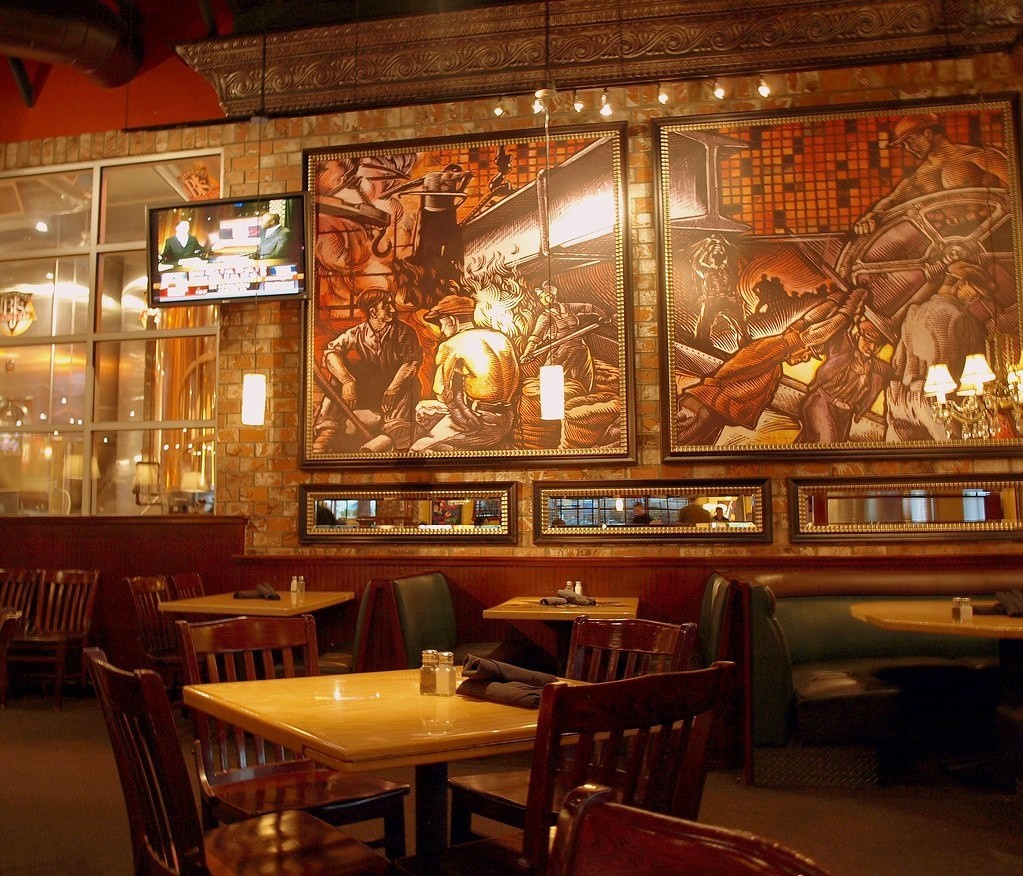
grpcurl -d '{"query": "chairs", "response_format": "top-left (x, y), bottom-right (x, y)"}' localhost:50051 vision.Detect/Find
top-left (0, 567), bottom-right (825, 876)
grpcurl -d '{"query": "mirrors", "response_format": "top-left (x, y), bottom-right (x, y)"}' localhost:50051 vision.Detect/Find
top-left (784, 474), bottom-right (1023, 544)
top-left (297, 482), bottom-right (518, 546)
top-left (532, 475), bottom-right (774, 544)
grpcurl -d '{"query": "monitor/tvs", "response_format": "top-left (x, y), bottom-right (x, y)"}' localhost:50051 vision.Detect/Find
top-left (144, 191), bottom-right (311, 309)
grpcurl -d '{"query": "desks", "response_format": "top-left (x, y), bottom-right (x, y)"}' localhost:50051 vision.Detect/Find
top-left (482, 597), bottom-right (640, 675)
top-left (158, 589), bottom-right (354, 683)
top-left (849, 598), bottom-right (1023, 793)
top-left (182, 667), bottom-right (694, 854)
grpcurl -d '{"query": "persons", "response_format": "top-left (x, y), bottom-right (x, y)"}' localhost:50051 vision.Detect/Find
top-left (713, 507), bottom-right (729, 521)
top-left (158, 220), bottom-right (209, 263)
top-left (317, 505), bottom-right (337, 524)
top-left (678, 499), bottom-right (711, 523)
top-left (634, 501), bottom-right (653, 523)
top-left (248, 212), bottom-right (292, 259)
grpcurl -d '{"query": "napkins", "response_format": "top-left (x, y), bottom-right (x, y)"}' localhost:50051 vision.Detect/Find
top-left (541, 589), bottom-right (597, 605)
top-left (233, 581), bottom-right (281, 600)
top-left (456, 653), bottom-right (561, 709)
top-left (969, 588), bottom-right (1023, 617)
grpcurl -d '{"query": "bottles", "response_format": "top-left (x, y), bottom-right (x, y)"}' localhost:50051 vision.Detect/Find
top-left (960, 598), bottom-right (972, 622)
top-left (419, 649), bottom-right (438, 696)
top-left (297, 576), bottom-right (305, 592)
top-left (566, 581), bottom-right (573, 591)
top-left (575, 581), bottom-right (582, 595)
top-left (291, 576), bottom-right (297, 592)
top-left (951, 597), bottom-right (961, 622)
top-left (436, 651), bottom-right (456, 696)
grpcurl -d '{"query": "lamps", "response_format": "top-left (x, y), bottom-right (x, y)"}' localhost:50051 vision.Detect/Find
top-left (540, 0), bottom-right (564, 421)
top-left (128, 461), bottom-right (210, 516)
top-left (241, 0), bottom-right (266, 426)
top-left (917, 0), bottom-right (1023, 440)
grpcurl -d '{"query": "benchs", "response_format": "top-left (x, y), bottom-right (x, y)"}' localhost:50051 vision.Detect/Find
top-left (641, 568), bottom-right (737, 769)
top-left (386, 568), bottom-right (504, 668)
top-left (734, 566), bottom-right (1023, 784)
top-left (275, 571), bottom-right (389, 676)
